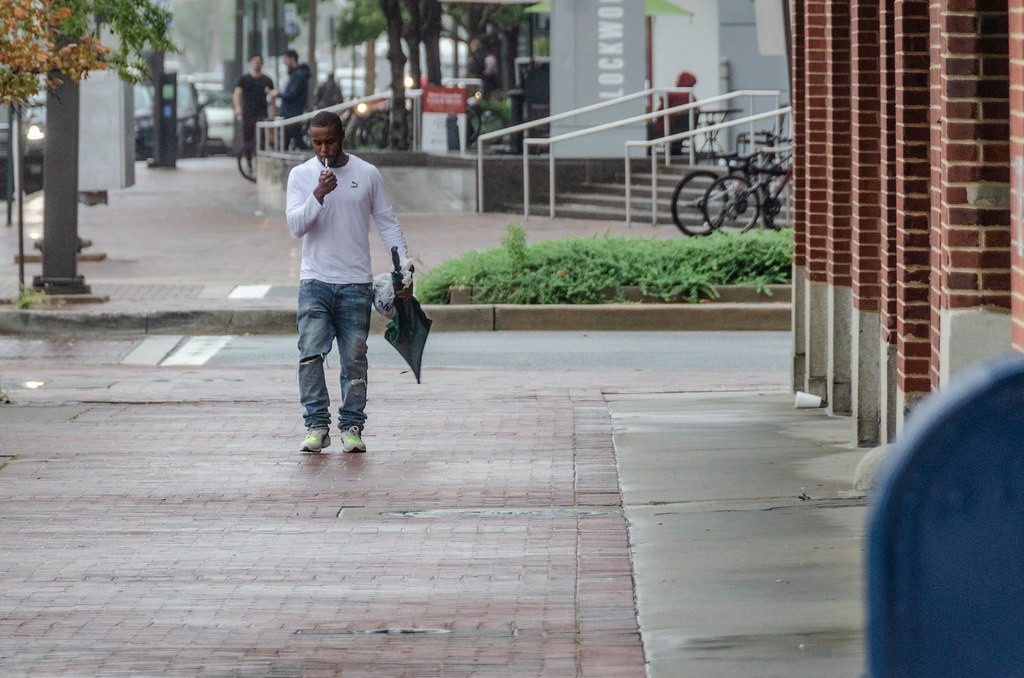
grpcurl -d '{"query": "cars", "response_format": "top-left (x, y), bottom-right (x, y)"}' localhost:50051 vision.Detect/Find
top-left (134, 83), bottom-right (154, 161)
top-left (175, 77), bottom-right (213, 157)
top-left (0, 73), bottom-right (46, 196)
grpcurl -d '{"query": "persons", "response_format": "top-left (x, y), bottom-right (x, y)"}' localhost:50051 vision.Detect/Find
top-left (287, 111), bottom-right (414, 454)
top-left (647, 72), bottom-right (699, 138)
top-left (270, 48), bottom-right (307, 150)
top-left (469, 37), bottom-right (496, 94)
top-left (314, 74), bottom-right (344, 110)
top-left (233, 53), bottom-right (275, 170)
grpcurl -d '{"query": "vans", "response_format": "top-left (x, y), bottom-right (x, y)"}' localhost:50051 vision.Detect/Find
top-left (195, 82), bottom-right (236, 152)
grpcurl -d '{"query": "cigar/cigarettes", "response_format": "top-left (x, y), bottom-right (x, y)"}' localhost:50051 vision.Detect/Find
top-left (325, 156), bottom-right (327, 169)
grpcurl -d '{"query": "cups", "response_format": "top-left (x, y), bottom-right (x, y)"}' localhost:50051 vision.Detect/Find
top-left (794, 389), bottom-right (823, 408)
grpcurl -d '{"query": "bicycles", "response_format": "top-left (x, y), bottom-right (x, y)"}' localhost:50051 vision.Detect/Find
top-left (238, 98), bottom-right (507, 182)
top-left (670, 131), bottom-right (793, 237)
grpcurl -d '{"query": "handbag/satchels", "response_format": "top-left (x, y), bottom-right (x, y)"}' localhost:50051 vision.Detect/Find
top-left (372, 257), bottom-right (413, 320)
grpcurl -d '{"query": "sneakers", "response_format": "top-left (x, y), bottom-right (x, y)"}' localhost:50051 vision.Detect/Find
top-left (299, 427), bottom-right (332, 453)
top-left (340, 426), bottom-right (366, 452)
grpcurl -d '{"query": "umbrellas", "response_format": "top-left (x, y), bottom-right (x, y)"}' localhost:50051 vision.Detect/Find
top-left (384, 244), bottom-right (432, 385)
top-left (526, 0), bottom-right (692, 18)
